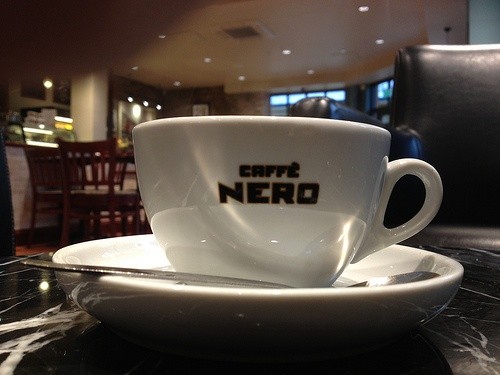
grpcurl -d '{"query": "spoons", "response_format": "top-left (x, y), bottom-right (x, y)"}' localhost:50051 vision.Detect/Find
top-left (21, 258), bottom-right (441, 289)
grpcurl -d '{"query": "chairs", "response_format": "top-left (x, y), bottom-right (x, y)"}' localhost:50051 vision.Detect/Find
top-left (388, 43), bottom-right (500, 256)
top-left (23, 138), bottom-right (149, 248)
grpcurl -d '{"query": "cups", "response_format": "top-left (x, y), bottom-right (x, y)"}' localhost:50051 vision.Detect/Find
top-left (133, 116), bottom-right (443, 289)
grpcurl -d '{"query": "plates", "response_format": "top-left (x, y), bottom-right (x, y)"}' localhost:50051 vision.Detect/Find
top-left (53, 234), bottom-right (463, 362)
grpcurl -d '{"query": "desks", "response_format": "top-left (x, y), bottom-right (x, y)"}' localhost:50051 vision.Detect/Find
top-left (0, 239), bottom-right (500, 375)
top-left (47, 155), bottom-right (135, 239)
